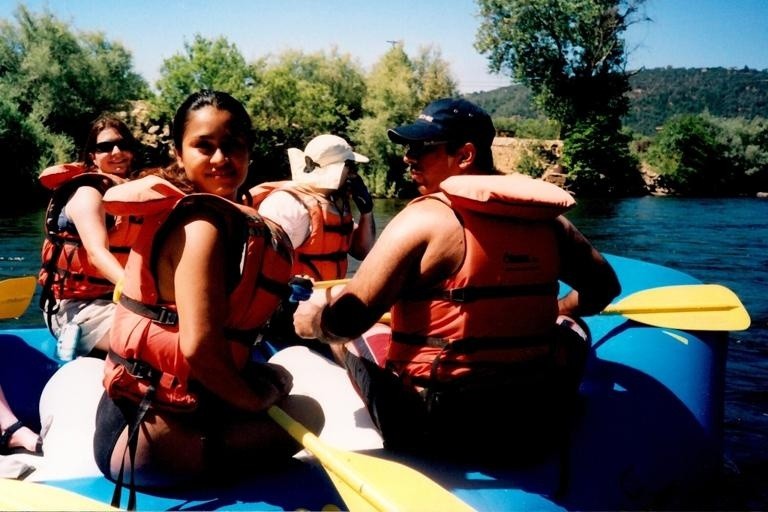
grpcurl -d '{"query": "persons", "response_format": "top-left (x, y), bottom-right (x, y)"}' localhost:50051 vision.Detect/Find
top-left (0, 384), bottom-right (43, 455)
top-left (38, 116), bottom-right (138, 353)
top-left (293, 99), bottom-right (621, 467)
top-left (258, 133), bottom-right (376, 282)
top-left (94, 89), bottom-right (294, 493)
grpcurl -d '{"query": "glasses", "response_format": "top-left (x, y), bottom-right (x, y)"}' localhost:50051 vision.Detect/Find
top-left (92, 137), bottom-right (132, 154)
top-left (409, 138), bottom-right (450, 153)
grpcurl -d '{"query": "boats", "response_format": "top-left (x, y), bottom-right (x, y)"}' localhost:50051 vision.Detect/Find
top-left (0, 251), bottom-right (726, 511)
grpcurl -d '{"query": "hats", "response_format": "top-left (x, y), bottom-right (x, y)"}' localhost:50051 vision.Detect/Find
top-left (387, 97), bottom-right (496, 148)
top-left (300, 134), bottom-right (370, 165)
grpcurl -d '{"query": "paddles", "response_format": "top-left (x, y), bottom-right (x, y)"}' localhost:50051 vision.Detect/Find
top-left (376, 284), bottom-right (752, 334)
top-left (266, 404), bottom-right (478, 512)
top-left (0, 276), bottom-right (38, 317)
